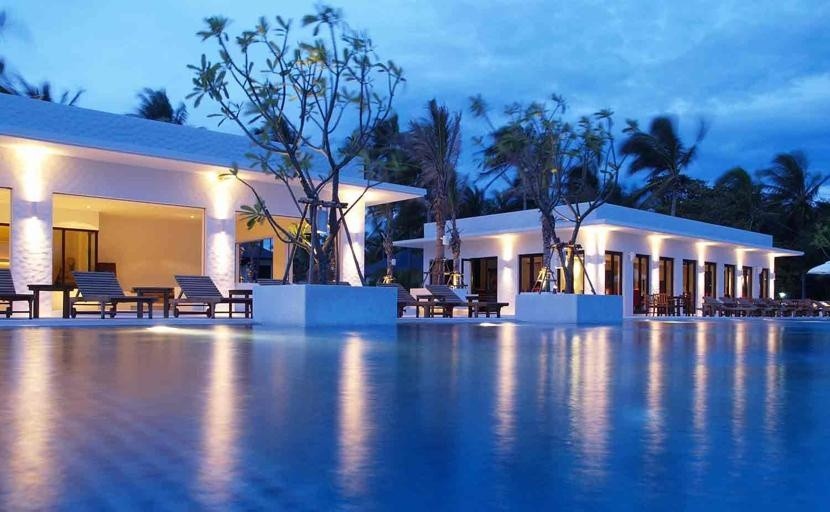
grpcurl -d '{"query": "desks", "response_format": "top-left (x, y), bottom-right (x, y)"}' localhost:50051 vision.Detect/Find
top-left (468, 303), bottom-right (509, 318)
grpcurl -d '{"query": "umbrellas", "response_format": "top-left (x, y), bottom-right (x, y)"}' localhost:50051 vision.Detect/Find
top-left (806, 261), bottom-right (830, 275)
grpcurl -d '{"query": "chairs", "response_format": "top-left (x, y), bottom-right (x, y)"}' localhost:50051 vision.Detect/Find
top-left (375, 283), bottom-right (471, 317)
top-left (641, 292), bottom-right (830, 319)
top-left (0, 266), bottom-right (254, 320)
